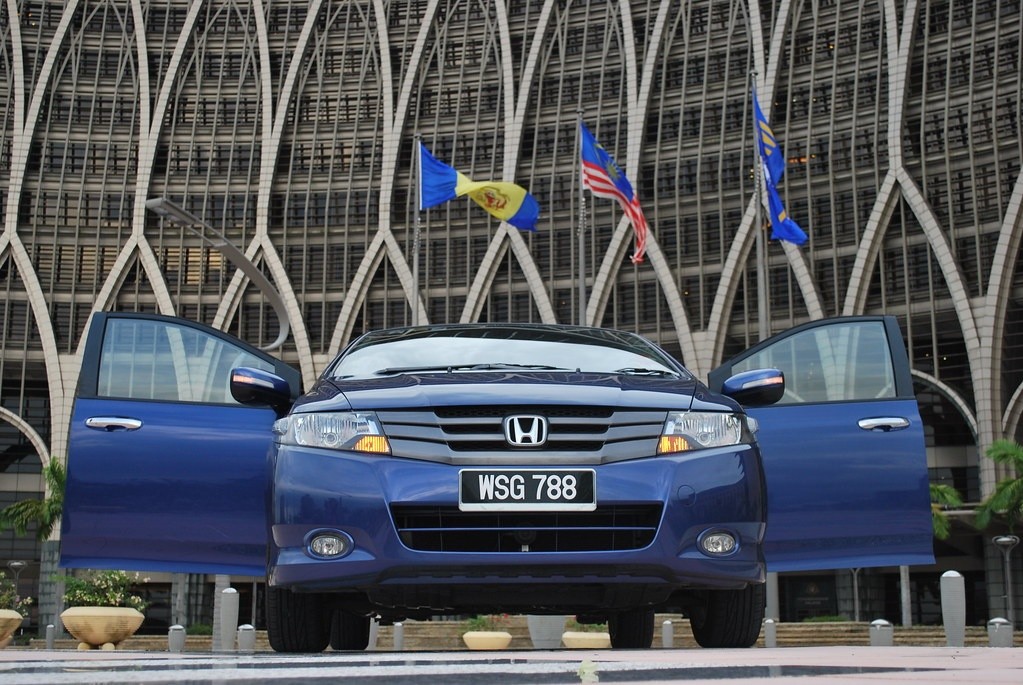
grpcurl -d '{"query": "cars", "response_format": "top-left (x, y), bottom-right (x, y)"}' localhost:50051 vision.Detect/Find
top-left (53, 308), bottom-right (946, 652)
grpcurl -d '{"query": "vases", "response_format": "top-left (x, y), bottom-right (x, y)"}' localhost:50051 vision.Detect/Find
top-left (561, 631), bottom-right (612, 649)
top-left (60, 607), bottom-right (145, 646)
top-left (0, 572), bottom-right (35, 618)
top-left (0, 609), bottom-right (25, 648)
top-left (462, 630), bottom-right (513, 650)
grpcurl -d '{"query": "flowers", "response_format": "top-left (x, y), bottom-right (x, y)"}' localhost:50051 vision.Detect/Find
top-left (564, 616), bottom-right (609, 632)
top-left (455, 613), bottom-right (512, 650)
top-left (50, 564), bottom-right (153, 615)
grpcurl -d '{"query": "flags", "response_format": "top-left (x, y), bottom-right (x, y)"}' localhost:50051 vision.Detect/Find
top-left (578, 120), bottom-right (646, 264)
top-left (417, 141), bottom-right (541, 233)
top-left (751, 82), bottom-right (809, 245)
